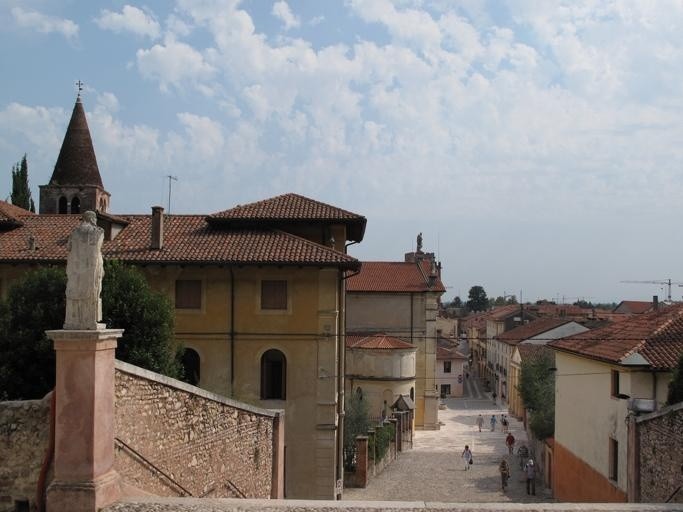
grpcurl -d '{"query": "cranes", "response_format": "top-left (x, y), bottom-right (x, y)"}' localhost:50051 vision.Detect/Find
top-left (617, 278), bottom-right (682, 301)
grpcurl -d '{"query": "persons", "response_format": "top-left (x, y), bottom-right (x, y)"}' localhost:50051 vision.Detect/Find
top-left (461, 413), bottom-right (536, 496)
